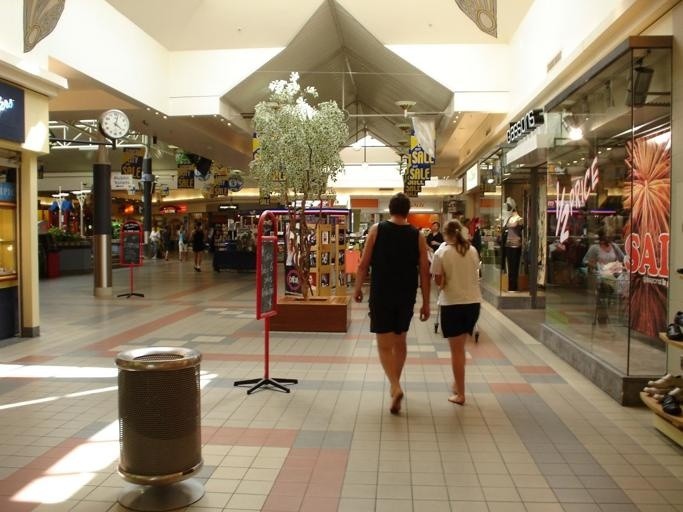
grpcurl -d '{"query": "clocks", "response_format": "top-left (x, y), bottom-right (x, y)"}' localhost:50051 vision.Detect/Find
top-left (97, 109), bottom-right (130, 140)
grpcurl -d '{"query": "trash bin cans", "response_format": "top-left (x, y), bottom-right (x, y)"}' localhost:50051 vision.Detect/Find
top-left (114, 347), bottom-right (206, 512)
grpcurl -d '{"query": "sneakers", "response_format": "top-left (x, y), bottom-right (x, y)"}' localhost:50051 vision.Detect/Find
top-left (667, 323), bottom-right (682, 339)
top-left (674, 311), bottom-right (683, 327)
top-left (643, 373), bottom-right (683, 416)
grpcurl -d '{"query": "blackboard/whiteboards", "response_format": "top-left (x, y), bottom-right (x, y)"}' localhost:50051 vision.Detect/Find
top-left (256, 236), bottom-right (277, 319)
top-left (120, 232), bottom-right (142, 265)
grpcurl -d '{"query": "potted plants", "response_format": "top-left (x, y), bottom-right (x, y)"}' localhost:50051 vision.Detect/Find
top-left (248, 69), bottom-right (352, 333)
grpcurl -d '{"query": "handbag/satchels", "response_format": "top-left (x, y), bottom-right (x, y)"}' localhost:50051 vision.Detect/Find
top-left (497, 229), bottom-right (508, 243)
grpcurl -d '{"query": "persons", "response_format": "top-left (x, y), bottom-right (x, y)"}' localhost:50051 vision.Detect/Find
top-left (149, 221), bottom-right (207, 272)
top-left (353, 193), bottom-right (483, 414)
top-left (580, 224), bottom-right (630, 334)
top-left (502, 196), bottom-right (524, 291)
